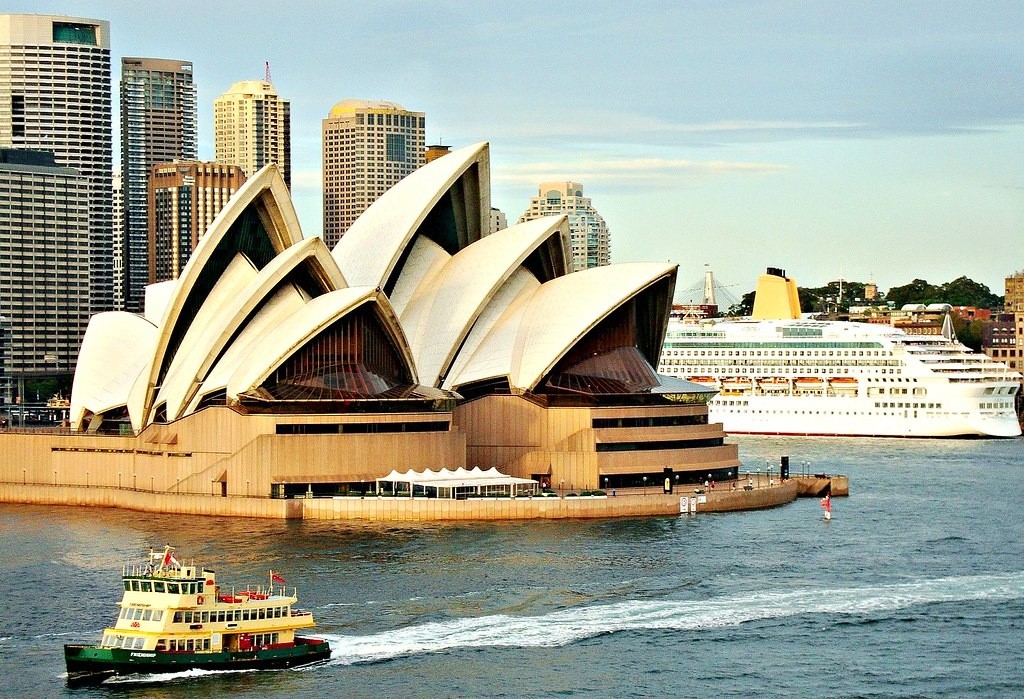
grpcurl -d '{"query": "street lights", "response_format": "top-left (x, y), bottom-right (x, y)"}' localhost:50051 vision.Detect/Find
top-left (708, 474), bottom-right (712, 493)
top-left (757, 468), bottom-right (761, 488)
top-left (133, 474), bottom-right (136, 490)
top-left (642, 476), bottom-right (648, 496)
top-left (23, 468), bottom-right (27, 486)
top-left (246, 479), bottom-right (250, 499)
top-left (766, 460), bottom-right (769, 477)
top-left (150, 476), bottom-right (154, 493)
top-left (281, 480), bottom-right (286, 499)
top-left (211, 478), bottom-right (215, 496)
top-left (360, 479), bottom-right (365, 500)
top-left (769, 465), bottom-right (774, 480)
top-left (604, 478), bottom-right (609, 497)
top-left (53, 470), bottom-right (58, 486)
top-left (85, 471), bottom-right (90, 487)
top-left (117, 472), bottom-right (122, 490)
top-left (779, 464), bottom-right (783, 480)
top-left (728, 472), bottom-right (732, 491)
top-left (746, 470), bottom-right (750, 486)
top-left (674, 475), bottom-right (680, 496)
top-left (561, 479), bottom-right (565, 500)
top-left (176, 477), bottom-right (180, 495)
top-left (802, 460), bottom-right (805, 479)
top-left (807, 461), bottom-right (810, 479)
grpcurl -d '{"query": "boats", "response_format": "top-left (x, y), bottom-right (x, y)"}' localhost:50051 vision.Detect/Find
top-left (64, 541), bottom-right (332, 677)
top-left (686, 376), bottom-right (859, 398)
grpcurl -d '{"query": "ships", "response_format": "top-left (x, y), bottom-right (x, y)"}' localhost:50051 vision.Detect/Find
top-left (656, 263), bottom-right (1023, 442)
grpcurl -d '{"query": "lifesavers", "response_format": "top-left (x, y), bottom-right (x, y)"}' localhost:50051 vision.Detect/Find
top-left (196, 595), bottom-right (204, 606)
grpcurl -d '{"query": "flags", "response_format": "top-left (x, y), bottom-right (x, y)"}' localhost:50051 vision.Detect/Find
top-left (272, 573), bottom-right (286, 583)
top-left (165, 554), bottom-right (172, 566)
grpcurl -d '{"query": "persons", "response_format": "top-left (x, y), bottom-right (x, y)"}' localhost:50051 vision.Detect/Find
top-left (710, 481), bottom-right (716, 492)
top-left (258, 640), bottom-right (264, 650)
top-left (732, 481), bottom-right (736, 492)
top-left (769, 477), bottom-right (774, 488)
top-left (698, 476), bottom-right (703, 485)
top-left (704, 480), bottom-right (709, 493)
top-left (542, 481), bottom-right (546, 494)
top-left (750, 478), bottom-right (753, 486)
